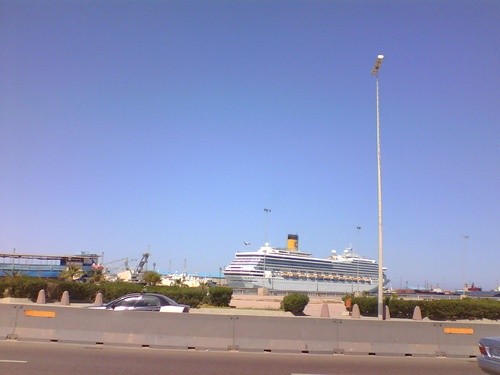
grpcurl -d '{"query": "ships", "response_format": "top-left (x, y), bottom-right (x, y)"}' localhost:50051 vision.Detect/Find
top-left (223, 232), bottom-right (391, 296)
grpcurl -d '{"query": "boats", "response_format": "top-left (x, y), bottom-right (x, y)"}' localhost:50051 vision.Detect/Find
top-left (454, 289), bottom-right (500, 297)
top-left (280, 271), bottom-right (372, 283)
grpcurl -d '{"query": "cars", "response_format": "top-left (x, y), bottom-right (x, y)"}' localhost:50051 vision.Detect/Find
top-left (476, 336), bottom-right (500, 374)
top-left (88, 292), bottom-right (191, 313)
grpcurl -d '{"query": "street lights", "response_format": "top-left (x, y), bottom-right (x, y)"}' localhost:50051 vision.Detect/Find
top-left (370, 54), bottom-right (384, 320)
